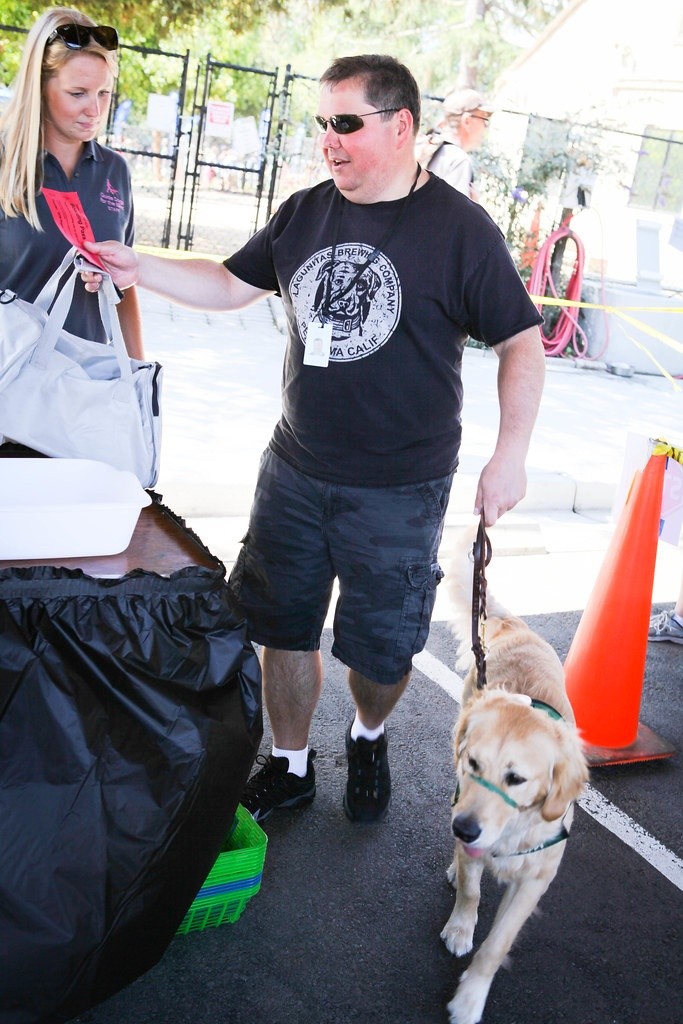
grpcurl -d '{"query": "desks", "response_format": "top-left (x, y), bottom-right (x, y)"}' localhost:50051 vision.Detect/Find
top-left (0, 489), bottom-right (227, 581)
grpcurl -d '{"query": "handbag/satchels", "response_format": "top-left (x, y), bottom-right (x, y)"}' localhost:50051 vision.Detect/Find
top-left (0, 246), bottom-right (163, 489)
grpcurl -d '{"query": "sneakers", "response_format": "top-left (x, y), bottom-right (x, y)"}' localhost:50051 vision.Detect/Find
top-left (343, 718), bottom-right (392, 826)
top-left (646, 611), bottom-right (683, 644)
top-left (228, 749), bottom-right (318, 829)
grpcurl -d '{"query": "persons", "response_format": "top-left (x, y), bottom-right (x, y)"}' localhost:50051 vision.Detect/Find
top-left (0, 8), bottom-right (147, 458)
top-left (77, 53), bottom-right (546, 824)
top-left (417, 88), bottom-right (495, 204)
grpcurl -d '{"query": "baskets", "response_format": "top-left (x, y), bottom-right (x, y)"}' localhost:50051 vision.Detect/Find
top-left (173, 801), bottom-right (268, 935)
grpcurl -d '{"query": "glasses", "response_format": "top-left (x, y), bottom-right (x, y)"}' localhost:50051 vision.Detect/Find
top-left (45, 25), bottom-right (119, 54)
top-left (315, 107), bottom-right (401, 136)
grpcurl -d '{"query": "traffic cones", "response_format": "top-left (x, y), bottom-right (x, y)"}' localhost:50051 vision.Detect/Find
top-left (561, 449), bottom-right (678, 769)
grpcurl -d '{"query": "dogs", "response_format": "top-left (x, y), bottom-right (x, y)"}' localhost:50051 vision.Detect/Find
top-left (436, 550), bottom-right (587, 1024)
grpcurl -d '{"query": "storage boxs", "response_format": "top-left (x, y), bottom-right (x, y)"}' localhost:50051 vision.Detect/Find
top-left (176, 796), bottom-right (270, 936)
